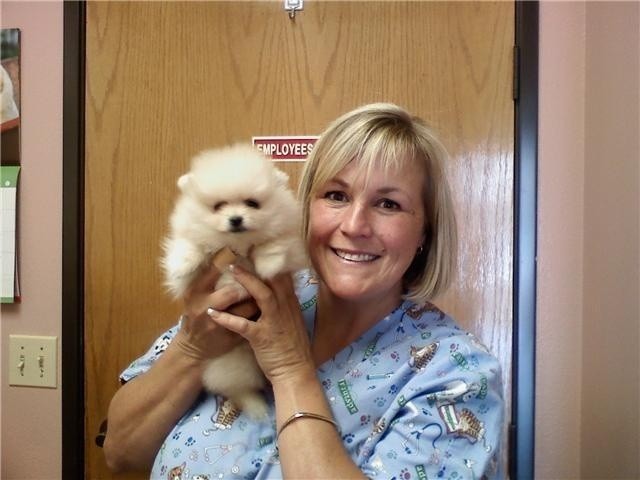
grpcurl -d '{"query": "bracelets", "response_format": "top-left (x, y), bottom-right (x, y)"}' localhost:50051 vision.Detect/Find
top-left (273, 408), bottom-right (342, 455)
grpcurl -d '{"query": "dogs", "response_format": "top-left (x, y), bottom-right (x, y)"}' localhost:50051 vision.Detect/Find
top-left (159, 143), bottom-right (306, 422)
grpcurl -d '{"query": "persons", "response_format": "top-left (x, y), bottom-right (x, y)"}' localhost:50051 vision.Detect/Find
top-left (96, 99), bottom-right (509, 480)
